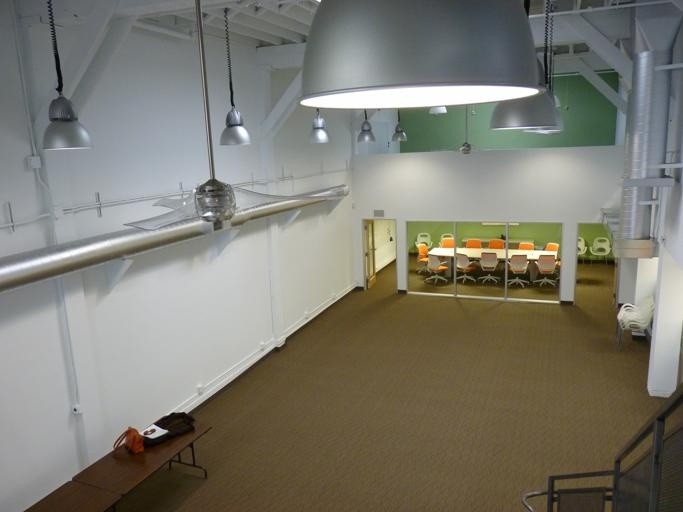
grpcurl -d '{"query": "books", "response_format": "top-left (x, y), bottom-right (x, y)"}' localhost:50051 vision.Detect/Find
top-left (138, 424), bottom-right (168, 440)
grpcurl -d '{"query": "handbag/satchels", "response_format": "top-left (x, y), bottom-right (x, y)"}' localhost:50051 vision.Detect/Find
top-left (125, 427), bottom-right (145, 453)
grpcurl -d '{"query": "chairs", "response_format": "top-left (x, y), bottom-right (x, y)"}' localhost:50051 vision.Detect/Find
top-left (577, 236), bottom-right (613, 265)
top-left (415, 232), bottom-right (561, 289)
top-left (616, 295), bottom-right (655, 351)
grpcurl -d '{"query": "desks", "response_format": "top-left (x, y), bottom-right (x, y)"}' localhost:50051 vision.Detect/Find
top-left (11, 420), bottom-right (213, 512)
